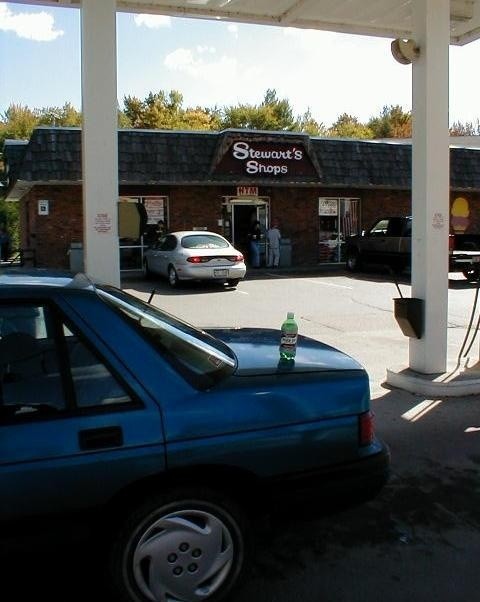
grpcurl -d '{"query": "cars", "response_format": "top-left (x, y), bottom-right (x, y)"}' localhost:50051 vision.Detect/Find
top-left (0, 270), bottom-right (392, 602)
top-left (144, 227), bottom-right (248, 293)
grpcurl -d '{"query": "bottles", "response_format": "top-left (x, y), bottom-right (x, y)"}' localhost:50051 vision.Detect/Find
top-left (278, 311), bottom-right (298, 361)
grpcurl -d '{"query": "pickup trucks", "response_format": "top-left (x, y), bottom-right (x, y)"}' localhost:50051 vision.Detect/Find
top-left (346, 212), bottom-right (480, 280)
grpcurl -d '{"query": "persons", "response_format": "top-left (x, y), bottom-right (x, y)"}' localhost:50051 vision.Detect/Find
top-left (248, 221), bottom-right (282, 269)
